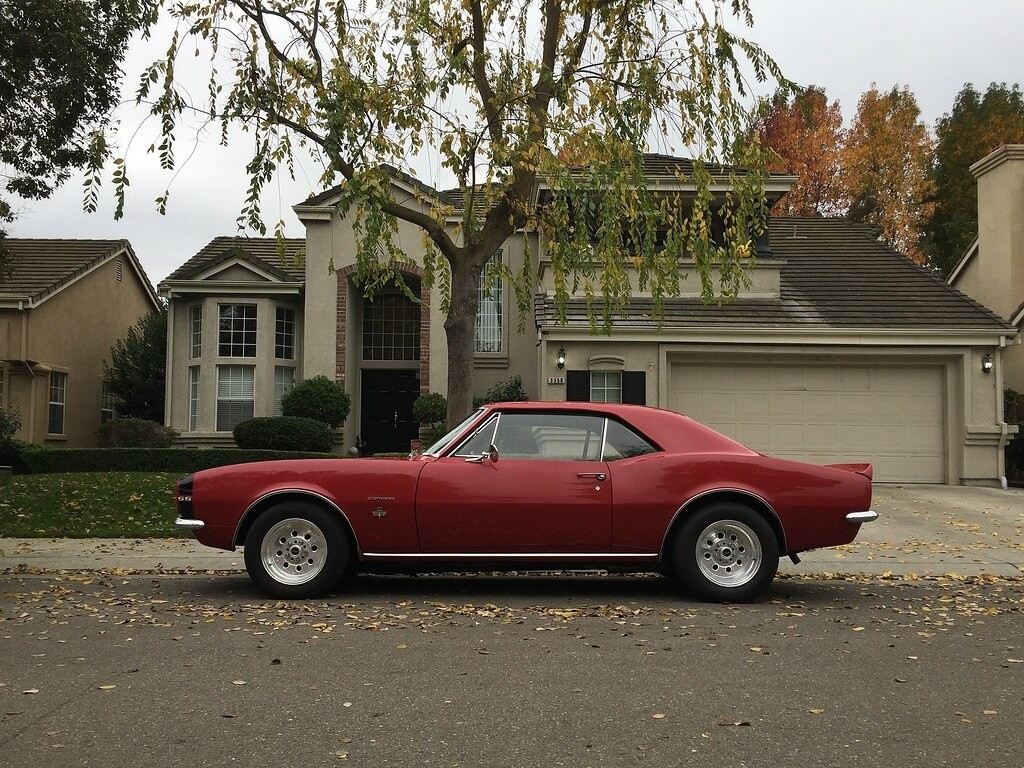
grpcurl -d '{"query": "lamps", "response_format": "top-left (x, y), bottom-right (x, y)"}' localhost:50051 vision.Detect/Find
top-left (982, 348), bottom-right (995, 373)
top-left (556, 344), bottom-right (567, 369)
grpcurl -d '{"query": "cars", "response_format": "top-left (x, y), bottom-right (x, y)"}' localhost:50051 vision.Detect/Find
top-left (171, 399), bottom-right (880, 609)
